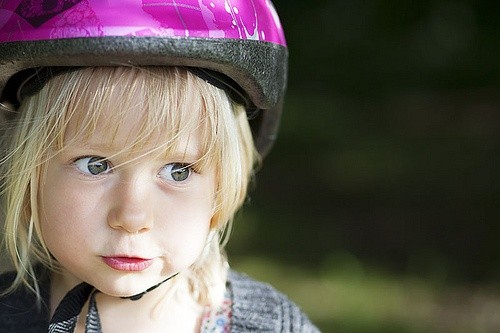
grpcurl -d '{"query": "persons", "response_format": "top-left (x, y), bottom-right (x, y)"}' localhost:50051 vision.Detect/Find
top-left (1, 0), bottom-right (321, 333)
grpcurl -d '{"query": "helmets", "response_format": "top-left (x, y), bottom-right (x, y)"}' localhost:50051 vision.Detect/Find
top-left (0, 0), bottom-right (288, 161)
